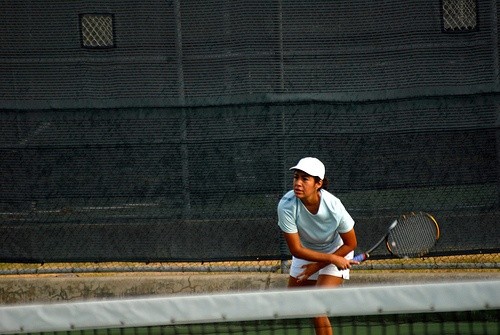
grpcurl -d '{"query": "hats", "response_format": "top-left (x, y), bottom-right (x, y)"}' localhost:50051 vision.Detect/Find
top-left (289, 157), bottom-right (326, 180)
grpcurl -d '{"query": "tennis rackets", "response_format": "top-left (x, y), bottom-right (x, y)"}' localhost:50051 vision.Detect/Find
top-left (337, 211), bottom-right (439, 272)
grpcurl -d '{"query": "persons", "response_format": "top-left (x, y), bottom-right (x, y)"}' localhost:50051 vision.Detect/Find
top-left (277, 157), bottom-right (357, 335)
